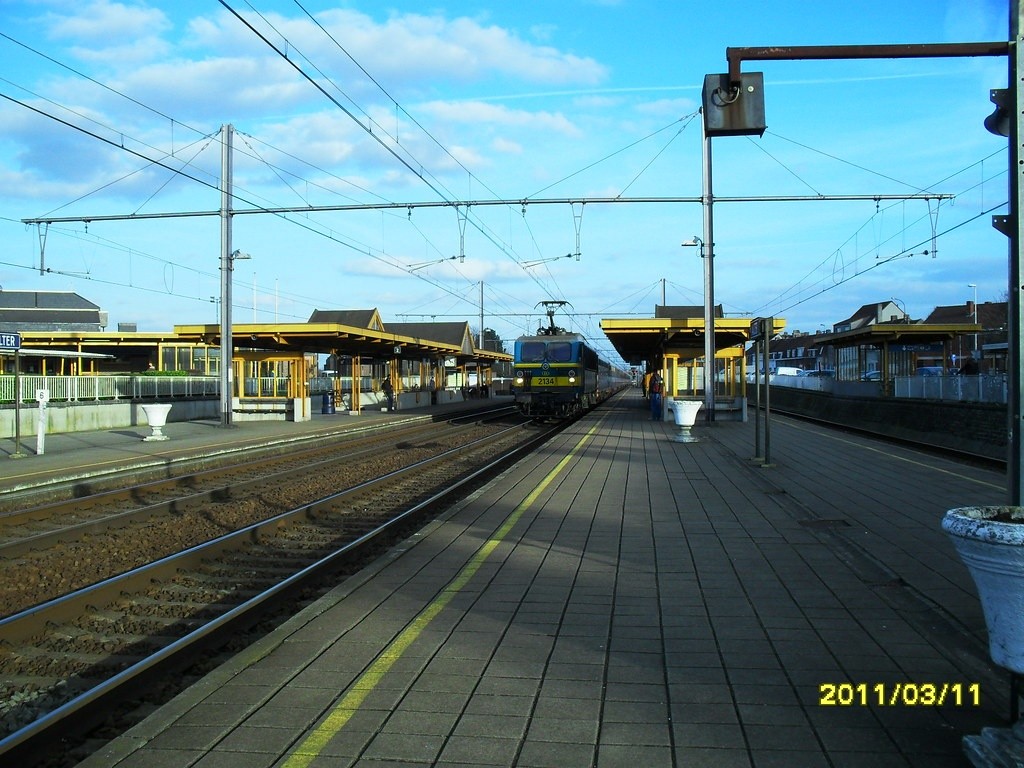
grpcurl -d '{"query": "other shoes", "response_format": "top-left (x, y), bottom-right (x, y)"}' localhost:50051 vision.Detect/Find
top-left (648, 417), bottom-right (657, 421)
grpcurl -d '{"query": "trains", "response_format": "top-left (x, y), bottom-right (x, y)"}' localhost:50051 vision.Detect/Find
top-left (512, 301), bottom-right (633, 422)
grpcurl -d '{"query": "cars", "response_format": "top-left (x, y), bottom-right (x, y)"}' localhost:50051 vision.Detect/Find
top-left (915, 366), bottom-right (961, 378)
top-left (849, 369), bottom-right (897, 382)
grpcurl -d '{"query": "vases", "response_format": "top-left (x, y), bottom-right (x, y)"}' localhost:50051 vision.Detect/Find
top-left (670, 399), bottom-right (703, 443)
top-left (942, 503), bottom-right (1024, 767)
top-left (140, 403), bottom-right (173, 442)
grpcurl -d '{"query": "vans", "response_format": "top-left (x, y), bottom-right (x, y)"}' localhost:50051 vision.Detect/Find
top-left (798, 370), bottom-right (835, 379)
top-left (321, 370), bottom-right (338, 380)
top-left (776, 366), bottom-right (803, 376)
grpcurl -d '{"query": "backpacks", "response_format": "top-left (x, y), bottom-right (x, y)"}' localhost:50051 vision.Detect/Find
top-left (652, 374), bottom-right (664, 394)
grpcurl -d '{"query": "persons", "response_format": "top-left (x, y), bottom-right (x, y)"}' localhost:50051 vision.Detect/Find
top-left (430, 376), bottom-right (435, 390)
top-left (384, 374), bottom-right (393, 411)
top-left (481, 382), bottom-right (488, 398)
top-left (641, 378), bottom-right (646, 397)
top-left (648, 370), bottom-right (663, 421)
top-left (956, 353), bottom-right (978, 403)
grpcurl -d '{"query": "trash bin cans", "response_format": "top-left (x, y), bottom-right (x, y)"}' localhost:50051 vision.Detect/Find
top-left (322, 391), bottom-right (335, 414)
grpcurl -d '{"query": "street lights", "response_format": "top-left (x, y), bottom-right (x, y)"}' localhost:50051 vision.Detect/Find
top-left (967, 284), bottom-right (977, 351)
